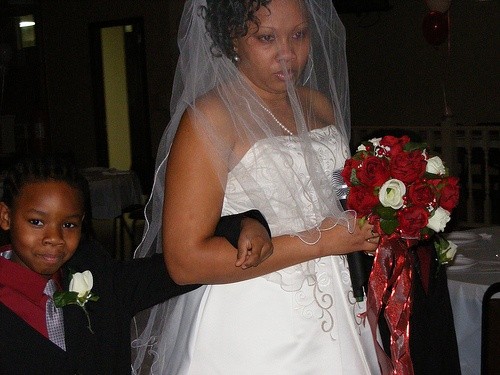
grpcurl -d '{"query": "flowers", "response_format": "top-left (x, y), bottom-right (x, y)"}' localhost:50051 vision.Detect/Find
top-left (53, 265), bottom-right (100, 336)
top-left (340, 135), bottom-right (461, 265)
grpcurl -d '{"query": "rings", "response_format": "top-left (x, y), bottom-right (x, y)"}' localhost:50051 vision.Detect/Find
top-left (367, 239), bottom-right (371, 242)
top-left (371, 231), bottom-right (374, 237)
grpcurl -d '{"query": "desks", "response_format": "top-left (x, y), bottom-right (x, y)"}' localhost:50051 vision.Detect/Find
top-left (80, 167), bottom-right (138, 260)
top-left (440, 226), bottom-right (500, 375)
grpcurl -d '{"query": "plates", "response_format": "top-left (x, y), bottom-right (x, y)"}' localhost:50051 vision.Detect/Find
top-left (447, 256), bottom-right (478, 273)
top-left (443, 233), bottom-right (479, 244)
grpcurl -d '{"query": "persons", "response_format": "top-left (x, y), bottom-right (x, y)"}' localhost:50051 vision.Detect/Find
top-left (129, 0), bottom-right (384, 375)
top-left (0, 156), bottom-right (274, 375)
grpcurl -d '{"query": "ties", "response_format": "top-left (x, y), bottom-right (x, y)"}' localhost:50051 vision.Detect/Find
top-left (42, 279), bottom-right (66, 351)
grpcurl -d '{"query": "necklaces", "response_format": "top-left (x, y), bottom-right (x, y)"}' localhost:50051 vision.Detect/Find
top-left (237, 74), bottom-right (294, 136)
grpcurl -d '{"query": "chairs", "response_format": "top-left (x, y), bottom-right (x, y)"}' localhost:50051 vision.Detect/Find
top-left (129, 204), bottom-right (145, 260)
top-left (480, 282), bottom-right (500, 375)
